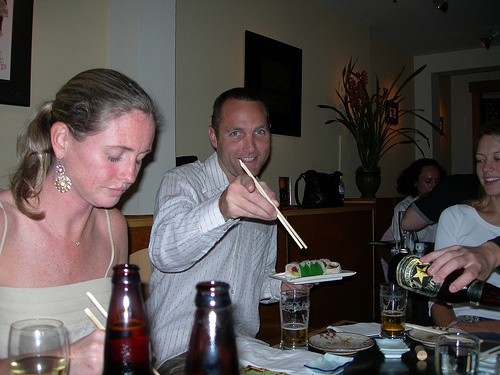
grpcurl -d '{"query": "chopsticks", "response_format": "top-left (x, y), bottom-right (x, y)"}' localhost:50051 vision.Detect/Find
top-left (239, 159), bottom-right (307, 250)
top-left (83, 291), bottom-right (160, 375)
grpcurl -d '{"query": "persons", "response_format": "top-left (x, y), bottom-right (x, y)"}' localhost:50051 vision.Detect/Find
top-left (142, 84), bottom-right (318, 374)
top-left (421, 235), bottom-right (499, 294)
top-left (400, 168), bottom-right (486, 240)
top-left (428, 119), bottom-right (500, 344)
top-left (0, 67), bottom-right (158, 375)
top-left (391, 158), bottom-right (446, 263)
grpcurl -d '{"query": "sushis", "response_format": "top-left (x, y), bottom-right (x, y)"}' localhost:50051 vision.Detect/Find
top-left (284, 258), bottom-right (341, 278)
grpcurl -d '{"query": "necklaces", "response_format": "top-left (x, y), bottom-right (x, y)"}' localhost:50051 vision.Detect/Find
top-left (45, 221), bottom-right (90, 245)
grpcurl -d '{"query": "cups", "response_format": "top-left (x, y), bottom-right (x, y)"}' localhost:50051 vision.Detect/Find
top-left (381, 283), bottom-right (407, 342)
top-left (280, 289), bottom-right (310, 351)
top-left (7, 318), bottom-right (69, 375)
top-left (434, 331), bottom-right (480, 375)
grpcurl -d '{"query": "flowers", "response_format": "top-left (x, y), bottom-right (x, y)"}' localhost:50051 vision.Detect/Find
top-left (316, 55), bottom-right (444, 168)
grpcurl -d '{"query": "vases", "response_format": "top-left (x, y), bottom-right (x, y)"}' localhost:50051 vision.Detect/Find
top-left (354, 166), bottom-right (383, 200)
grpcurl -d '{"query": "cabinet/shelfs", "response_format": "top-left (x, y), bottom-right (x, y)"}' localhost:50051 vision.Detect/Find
top-left (275, 197), bottom-right (406, 273)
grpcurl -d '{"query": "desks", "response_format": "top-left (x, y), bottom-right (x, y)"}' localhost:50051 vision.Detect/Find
top-left (156, 317), bottom-right (500, 375)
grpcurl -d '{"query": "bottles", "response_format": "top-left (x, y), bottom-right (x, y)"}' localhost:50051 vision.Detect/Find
top-left (182, 280), bottom-right (240, 375)
top-left (387, 252), bottom-right (500, 307)
top-left (102, 263), bottom-right (153, 375)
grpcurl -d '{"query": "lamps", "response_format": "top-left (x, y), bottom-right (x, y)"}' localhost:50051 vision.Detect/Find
top-left (433, 0), bottom-right (451, 14)
top-left (480, 32), bottom-right (500, 50)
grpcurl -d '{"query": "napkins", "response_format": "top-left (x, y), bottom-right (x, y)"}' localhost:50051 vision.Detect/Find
top-left (328, 322), bottom-right (386, 339)
top-left (236, 333), bottom-right (352, 375)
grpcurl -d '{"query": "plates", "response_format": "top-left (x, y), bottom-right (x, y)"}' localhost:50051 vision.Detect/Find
top-left (407, 325), bottom-right (469, 347)
top-left (376, 338), bottom-right (410, 359)
top-left (268, 270), bottom-right (357, 285)
top-left (307, 331), bottom-right (374, 356)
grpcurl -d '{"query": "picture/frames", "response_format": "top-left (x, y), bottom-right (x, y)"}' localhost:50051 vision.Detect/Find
top-left (386, 101), bottom-right (401, 126)
top-left (0, 0), bottom-right (36, 111)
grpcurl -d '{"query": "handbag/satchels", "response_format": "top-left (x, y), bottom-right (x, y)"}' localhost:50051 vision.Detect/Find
top-left (294, 170), bottom-right (345, 209)
